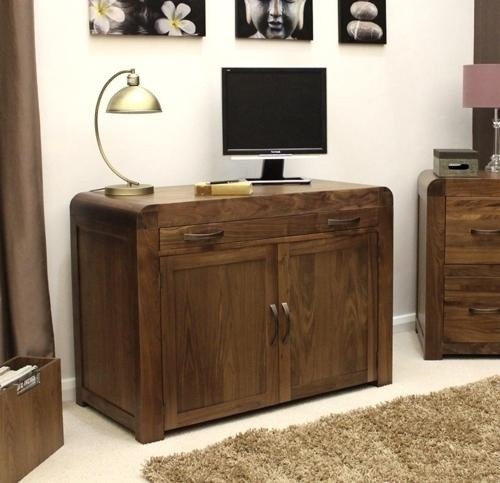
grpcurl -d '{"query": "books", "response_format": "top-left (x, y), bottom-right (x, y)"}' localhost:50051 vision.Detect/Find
top-left (0, 363), bottom-right (39, 391)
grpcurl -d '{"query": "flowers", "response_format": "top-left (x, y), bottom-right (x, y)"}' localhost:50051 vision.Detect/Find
top-left (89, 0), bottom-right (124, 32)
top-left (155, 1), bottom-right (194, 36)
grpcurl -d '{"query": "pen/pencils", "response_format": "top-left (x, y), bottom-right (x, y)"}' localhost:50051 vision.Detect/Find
top-left (206, 180), bottom-right (240, 184)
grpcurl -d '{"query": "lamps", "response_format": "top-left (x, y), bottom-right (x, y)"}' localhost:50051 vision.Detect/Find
top-left (462, 64), bottom-right (499, 169)
top-left (93, 67), bottom-right (166, 195)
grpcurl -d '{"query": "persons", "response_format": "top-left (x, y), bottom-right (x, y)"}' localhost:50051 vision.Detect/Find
top-left (243, 0), bottom-right (306, 40)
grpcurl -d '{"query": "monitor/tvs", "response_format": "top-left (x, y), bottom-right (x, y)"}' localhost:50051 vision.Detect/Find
top-left (221, 67), bottom-right (327, 184)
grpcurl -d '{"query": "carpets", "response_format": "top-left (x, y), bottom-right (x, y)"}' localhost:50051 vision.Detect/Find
top-left (143, 372), bottom-right (500, 482)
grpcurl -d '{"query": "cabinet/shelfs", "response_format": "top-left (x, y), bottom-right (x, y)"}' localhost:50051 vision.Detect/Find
top-left (415, 170), bottom-right (499, 359)
top-left (67, 180), bottom-right (393, 442)
